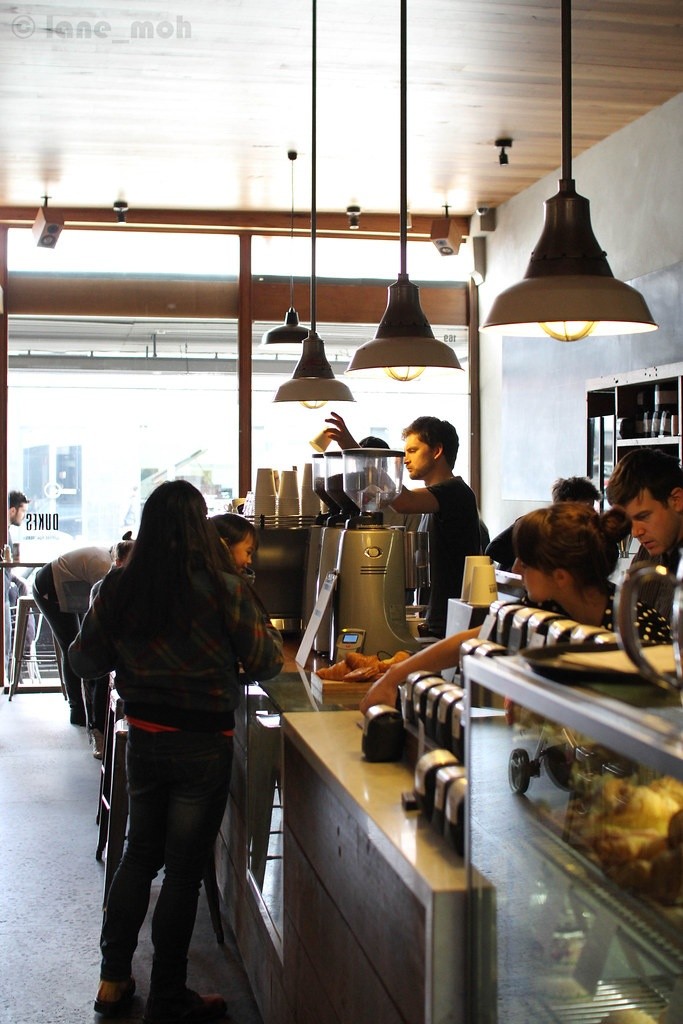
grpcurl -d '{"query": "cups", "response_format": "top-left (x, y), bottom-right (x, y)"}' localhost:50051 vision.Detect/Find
top-left (460, 555), bottom-right (491, 602)
top-left (254, 463), bottom-right (321, 528)
top-left (467, 566), bottom-right (498, 605)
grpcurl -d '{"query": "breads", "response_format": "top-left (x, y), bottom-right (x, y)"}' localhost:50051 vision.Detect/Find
top-left (567, 769), bottom-right (683, 909)
top-left (315, 650), bottom-right (410, 683)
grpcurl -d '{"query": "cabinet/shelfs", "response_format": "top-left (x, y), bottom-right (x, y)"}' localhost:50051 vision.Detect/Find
top-left (579, 363), bottom-right (683, 555)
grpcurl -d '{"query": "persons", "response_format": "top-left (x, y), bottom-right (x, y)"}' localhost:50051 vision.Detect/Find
top-left (359, 503), bottom-right (671, 727)
top-left (484, 446), bottom-right (682, 717)
top-left (0, 492), bottom-right (30, 705)
top-left (322, 411), bottom-right (495, 648)
top-left (33, 481), bottom-right (284, 1024)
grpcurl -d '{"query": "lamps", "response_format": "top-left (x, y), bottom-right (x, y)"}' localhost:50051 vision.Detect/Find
top-left (260, 154), bottom-right (319, 344)
top-left (476, 0), bottom-right (661, 341)
top-left (347, 0), bottom-right (463, 381)
top-left (347, 206), bottom-right (361, 230)
top-left (114, 202), bottom-right (128, 225)
top-left (495, 138), bottom-right (512, 165)
top-left (273, 0), bottom-right (358, 411)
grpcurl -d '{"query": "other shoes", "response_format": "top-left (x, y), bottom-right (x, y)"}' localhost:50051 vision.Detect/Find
top-left (87, 729), bottom-right (105, 759)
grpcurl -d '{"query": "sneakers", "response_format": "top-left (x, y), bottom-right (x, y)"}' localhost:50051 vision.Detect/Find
top-left (94, 976), bottom-right (144, 1019)
top-left (142, 980), bottom-right (228, 1024)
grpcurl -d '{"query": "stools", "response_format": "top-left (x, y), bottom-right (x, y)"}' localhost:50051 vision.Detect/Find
top-left (8, 595), bottom-right (70, 701)
top-left (96, 670), bottom-right (227, 947)
